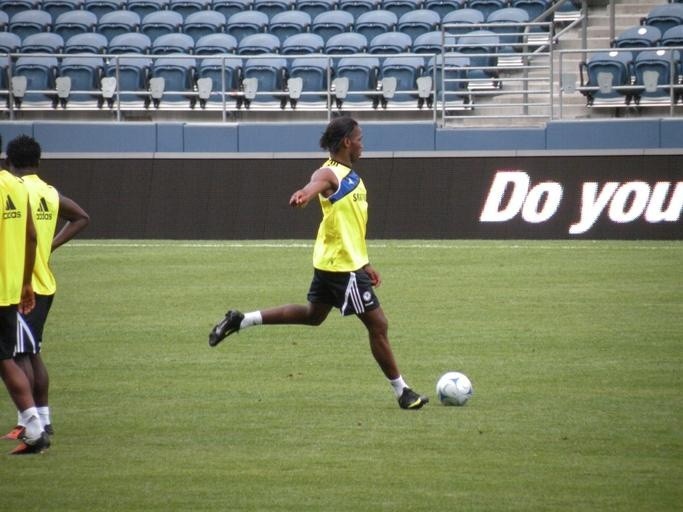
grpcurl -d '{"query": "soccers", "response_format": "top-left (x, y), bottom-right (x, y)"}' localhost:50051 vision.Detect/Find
top-left (437, 372), bottom-right (471, 405)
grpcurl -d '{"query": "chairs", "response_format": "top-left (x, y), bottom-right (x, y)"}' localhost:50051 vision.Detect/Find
top-left (578, 2), bottom-right (683, 113)
top-left (1, 1), bottom-right (593, 113)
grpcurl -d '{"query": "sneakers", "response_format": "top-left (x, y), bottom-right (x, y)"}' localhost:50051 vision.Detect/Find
top-left (209, 310), bottom-right (244, 347)
top-left (0, 423), bottom-right (53, 454)
top-left (398, 387), bottom-right (428, 409)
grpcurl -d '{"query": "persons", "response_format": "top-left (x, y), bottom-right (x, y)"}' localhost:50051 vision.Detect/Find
top-left (0, 135), bottom-right (52, 455)
top-left (208, 116), bottom-right (430, 411)
top-left (0, 134), bottom-right (90, 442)
